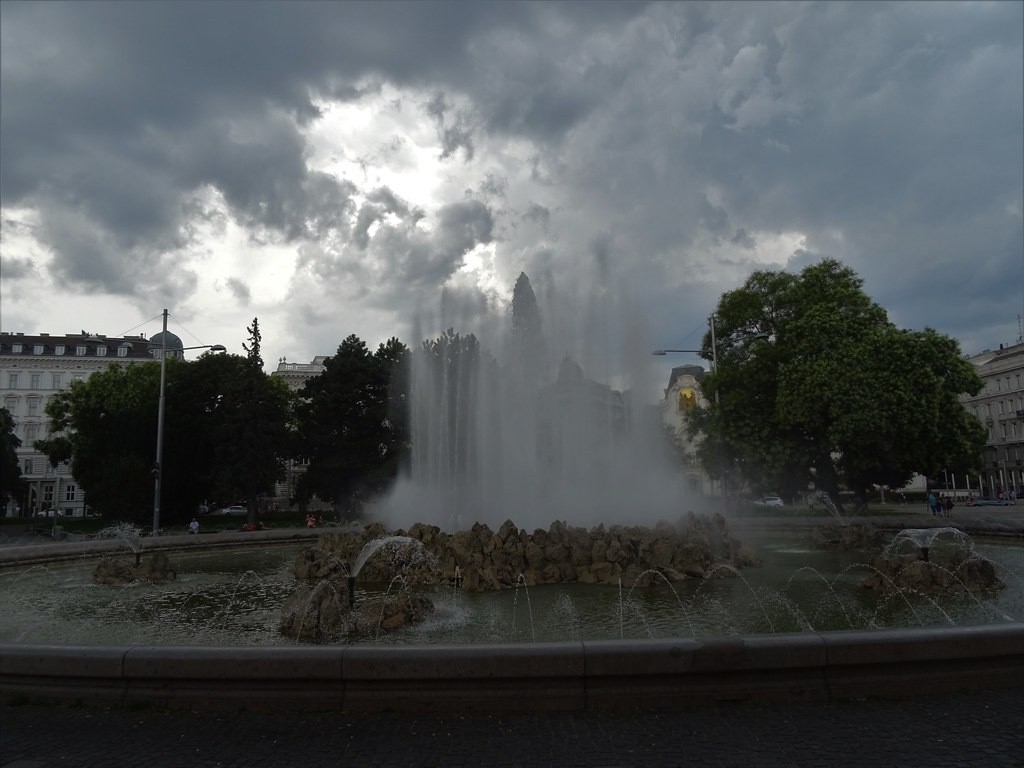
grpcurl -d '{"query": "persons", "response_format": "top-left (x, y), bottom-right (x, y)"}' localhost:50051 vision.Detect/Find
top-left (190, 518), bottom-right (199, 534)
top-left (929, 494), bottom-right (955, 517)
top-left (307, 515), bottom-right (323, 527)
top-left (242, 517), bottom-right (262, 531)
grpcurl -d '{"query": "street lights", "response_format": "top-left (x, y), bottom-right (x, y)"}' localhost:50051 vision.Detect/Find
top-left (84, 309), bottom-right (226, 536)
top-left (650, 312), bottom-right (770, 422)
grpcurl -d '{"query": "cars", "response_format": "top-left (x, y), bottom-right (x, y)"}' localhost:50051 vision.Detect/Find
top-left (756, 496), bottom-right (785, 512)
top-left (967, 495), bottom-right (1013, 507)
top-left (37, 507), bottom-right (65, 519)
top-left (222, 506), bottom-right (249, 518)
